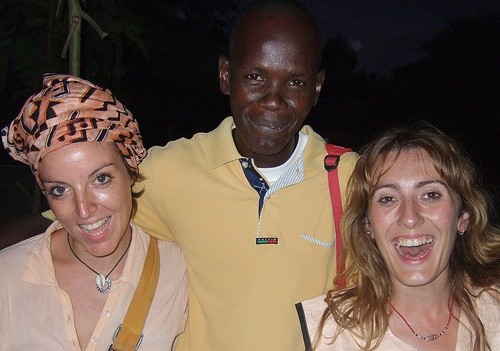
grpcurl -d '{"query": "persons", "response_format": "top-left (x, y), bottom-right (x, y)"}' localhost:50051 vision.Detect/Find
top-left (0, 0), bottom-right (364, 351)
top-left (294, 126), bottom-right (500, 351)
top-left (0, 73), bottom-right (190, 351)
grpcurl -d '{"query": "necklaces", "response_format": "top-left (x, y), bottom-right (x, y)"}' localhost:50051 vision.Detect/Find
top-left (388, 294), bottom-right (454, 341)
top-left (67, 227), bottom-right (132, 294)
